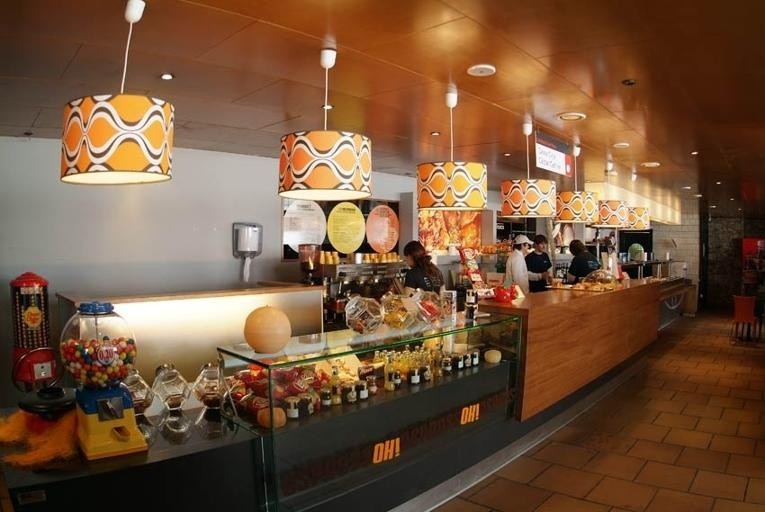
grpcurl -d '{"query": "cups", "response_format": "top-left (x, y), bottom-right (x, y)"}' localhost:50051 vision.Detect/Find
top-left (319, 250), bottom-right (340, 265)
top-left (354, 251), bottom-right (400, 264)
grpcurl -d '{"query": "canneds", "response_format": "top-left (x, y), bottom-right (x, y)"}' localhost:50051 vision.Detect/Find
top-left (284, 396), bottom-right (301, 421)
top-left (320, 390), bottom-right (331, 408)
top-left (420, 366), bottom-right (431, 382)
top-left (298, 393), bottom-right (312, 416)
top-left (343, 383), bottom-right (357, 404)
top-left (408, 369), bottom-right (420, 385)
top-left (441, 352), bottom-right (481, 374)
top-left (354, 381), bottom-right (368, 401)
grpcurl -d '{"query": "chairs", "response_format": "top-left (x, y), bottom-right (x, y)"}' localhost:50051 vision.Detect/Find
top-left (731, 292), bottom-right (758, 343)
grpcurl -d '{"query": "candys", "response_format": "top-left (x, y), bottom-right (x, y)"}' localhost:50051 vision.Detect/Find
top-left (60, 335), bottom-right (136, 389)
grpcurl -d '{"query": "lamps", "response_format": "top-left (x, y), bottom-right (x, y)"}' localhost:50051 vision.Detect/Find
top-left (622, 170), bottom-right (649, 231)
top-left (587, 151), bottom-right (631, 228)
top-left (416, 80), bottom-right (490, 212)
top-left (276, 42), bottom-right (375, 208)
top-left (56, 2), bottom-right (177, 188)
top-left (557, 146), bottom-right (603, 226)
top-left (499, 119), bottom-right (558, 221)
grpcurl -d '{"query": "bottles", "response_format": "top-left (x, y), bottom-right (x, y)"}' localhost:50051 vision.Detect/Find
top-left (132, 406), bottom-right (228, 447)
top-left (329, 366), bottom-right (341, 405)
top-left (123, 360), bottom-right (222, 415)
top-left (555, 263), bottom-right (570, 283)
top-left (508, 232), bottom-right (516, 250)
top-left (742, 240), bottom-right (765, 316)
top-left (373, 344), bottom-right (444, 392)
top-left (343, 288), bottom-right (448, 336)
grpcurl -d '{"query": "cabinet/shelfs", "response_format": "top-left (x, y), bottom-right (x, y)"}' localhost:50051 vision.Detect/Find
top-left (218, 319), bottom-right (516, 512)
top-left (1, 401), bottom-right (269, 511)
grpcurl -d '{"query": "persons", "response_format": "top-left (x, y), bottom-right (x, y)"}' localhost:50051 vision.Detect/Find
top-left (568, 240), bottom-right (602, 284)
top-left (504, 235), bottom-right (550, 295)
top-left (395, 241), bottom-right (445, 297)
top-left (525, 235), bottom-right (553, 293)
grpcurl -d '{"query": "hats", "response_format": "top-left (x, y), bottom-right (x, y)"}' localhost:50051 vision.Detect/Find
top-left (513, 234), bottom-right (534, 245)
